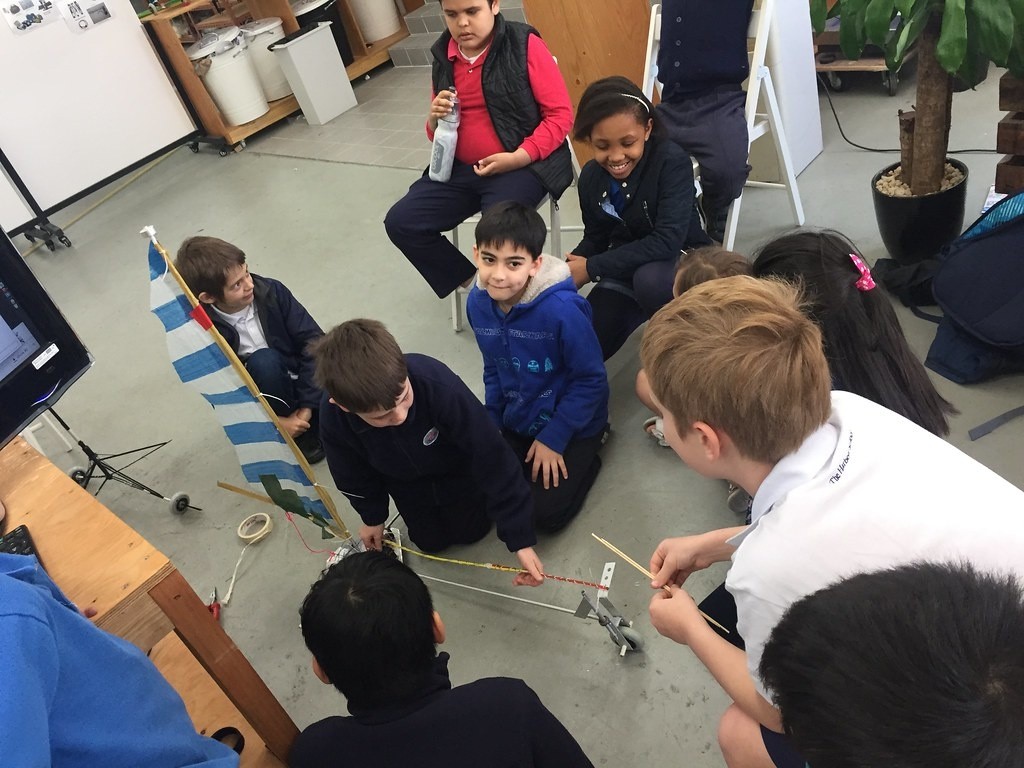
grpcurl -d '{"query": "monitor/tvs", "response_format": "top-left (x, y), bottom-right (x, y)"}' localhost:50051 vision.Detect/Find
top-left (0, 225), bottom-right (95, 453)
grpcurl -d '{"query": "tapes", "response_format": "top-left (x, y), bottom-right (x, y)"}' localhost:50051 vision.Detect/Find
top-left (238, 512), bottom-right (273, 546)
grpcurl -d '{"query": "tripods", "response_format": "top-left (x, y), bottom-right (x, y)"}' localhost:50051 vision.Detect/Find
top-left (47, 405), bottom-right (203, 515)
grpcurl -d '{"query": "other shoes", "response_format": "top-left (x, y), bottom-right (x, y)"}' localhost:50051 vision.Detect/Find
top-left (601, 419), bottom-right (612, 446)
top-left (294, 428), bottom-right (325, 464)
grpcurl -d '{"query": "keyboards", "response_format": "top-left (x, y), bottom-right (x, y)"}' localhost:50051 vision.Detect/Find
top-left (0, 524), bottom-right (46, 575)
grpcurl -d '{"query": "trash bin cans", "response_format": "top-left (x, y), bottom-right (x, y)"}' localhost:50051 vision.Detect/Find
top-left (266, 17), bottom-right (358, 127)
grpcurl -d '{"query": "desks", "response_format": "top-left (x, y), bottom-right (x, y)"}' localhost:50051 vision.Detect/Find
top-left (0, 434), bottom-right (302, 768)
top-left (139, 0), bottom-right (410, 157)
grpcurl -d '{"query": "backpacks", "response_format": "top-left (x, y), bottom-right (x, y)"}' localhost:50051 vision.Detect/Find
top-left (872, 186), bottom-right (1024, 384)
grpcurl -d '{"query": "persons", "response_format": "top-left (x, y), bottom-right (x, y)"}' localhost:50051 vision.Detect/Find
top-left (175, 238), bottom-right (342, 455)
top-left (0, 548), bottom-right (242, 768)
top-left (286, 547), bottom-right (594, 768)
top-left (645, 228), bottom-right (959, 531)
top-left (563, 73), bottom-right (714, 361)
top-left (386, 0), bottom-right (574, 301)
top-left (318, 318), bottom-right (549, 589)
top-left (461, 205), bottom-right (617, 543)
top-left (654, 0), bottom-right (757, 253)
top-left (635, 275), bottom-right (1024, 767)
top-left (753, 556), bottom-right (1023, 767)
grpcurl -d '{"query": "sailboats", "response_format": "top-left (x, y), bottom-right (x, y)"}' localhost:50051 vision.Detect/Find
top-left (139, 224), bottom-right (404, 571)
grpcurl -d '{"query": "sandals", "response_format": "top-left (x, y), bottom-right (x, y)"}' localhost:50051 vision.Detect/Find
top-left (643, 416), bottom-right (672, 445)
top-left (728, 481), bottom-right (751, 513)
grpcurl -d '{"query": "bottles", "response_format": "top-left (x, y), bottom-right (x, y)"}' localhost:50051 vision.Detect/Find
top-left (429, 86), bottom-right (460, 182)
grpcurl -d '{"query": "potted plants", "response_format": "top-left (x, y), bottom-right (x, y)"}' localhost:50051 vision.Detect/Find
top-left (809, 0), bottom-right (1024, 265)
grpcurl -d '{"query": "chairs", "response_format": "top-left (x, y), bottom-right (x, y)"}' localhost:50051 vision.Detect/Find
top-left (451, 54), bottom-right (583, 331)
top-left (644, 0), bottom-right (806, 253)
